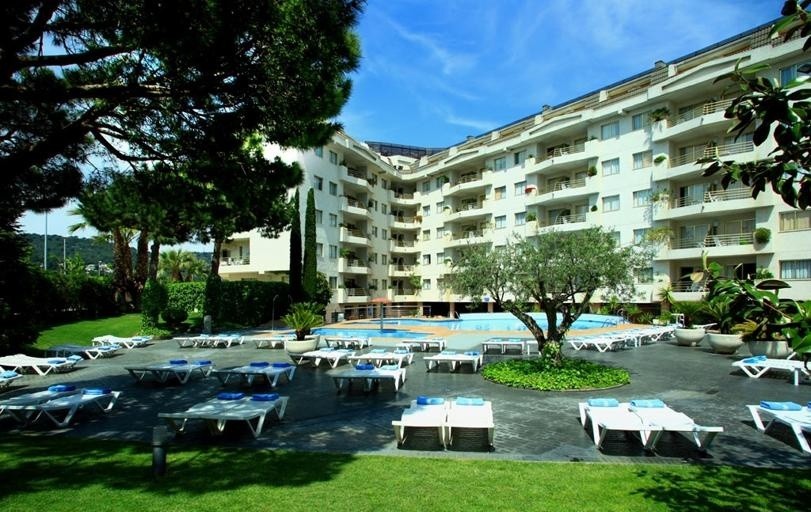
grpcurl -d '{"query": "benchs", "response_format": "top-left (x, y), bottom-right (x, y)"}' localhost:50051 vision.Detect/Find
top-left (0, 311), bottom-right (811, 459)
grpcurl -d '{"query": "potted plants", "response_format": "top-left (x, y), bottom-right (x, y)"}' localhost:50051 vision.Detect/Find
top-left (707, 279), bottom-right (743, 351)
top-left (745, 287), bottom-right (790, 358)
top-left (672, 298), bottom-right (706, 345)
